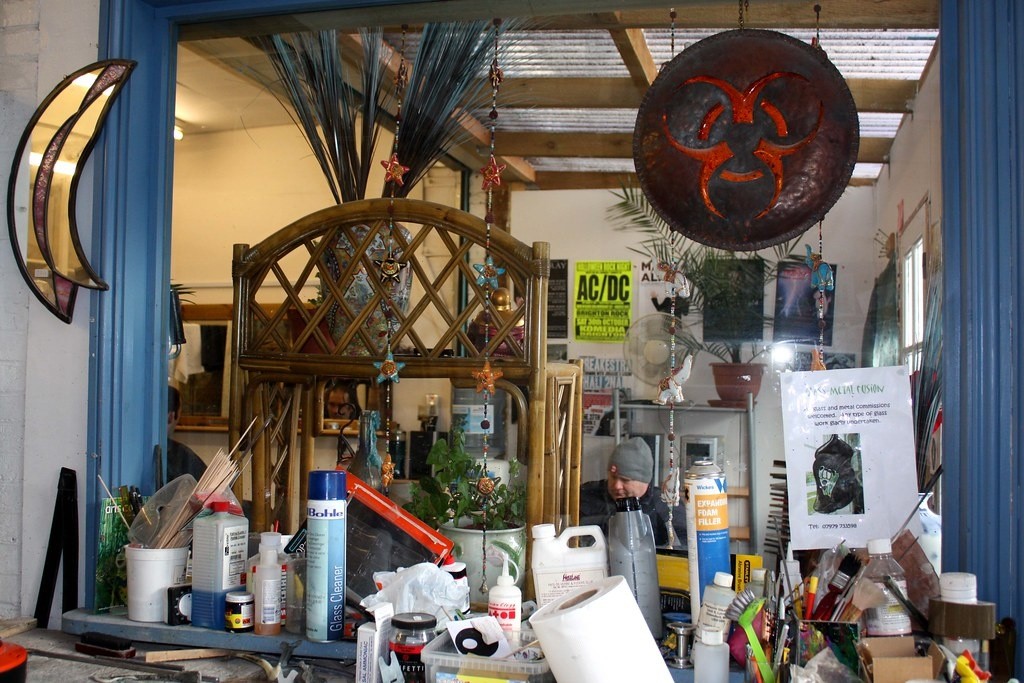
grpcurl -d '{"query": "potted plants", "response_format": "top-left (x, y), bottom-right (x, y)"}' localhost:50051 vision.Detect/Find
top-left (406, 424), bottom-right (527, 608)
top-left (603, 172), bottom-right (805, 401)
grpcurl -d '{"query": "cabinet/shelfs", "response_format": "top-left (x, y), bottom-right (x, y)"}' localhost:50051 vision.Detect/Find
top-left (610, 386), bottom-right (759, 556)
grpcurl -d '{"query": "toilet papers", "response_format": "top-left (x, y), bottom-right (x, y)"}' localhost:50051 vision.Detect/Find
top-left (528, 574), bottom-right (675, 683)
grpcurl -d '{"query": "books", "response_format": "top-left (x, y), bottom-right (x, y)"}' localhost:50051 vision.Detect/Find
top-left (93, 496), bottom-right (149, 615)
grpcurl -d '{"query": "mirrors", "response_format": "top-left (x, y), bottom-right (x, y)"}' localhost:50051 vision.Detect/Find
top-left (169, 301), bottom-right (393, 438)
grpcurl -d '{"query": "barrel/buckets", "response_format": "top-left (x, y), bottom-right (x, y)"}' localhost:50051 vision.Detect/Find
top-left (531, 523), bottom-right (610, 611)
top-left (124, 543), bottom-right (190, 623)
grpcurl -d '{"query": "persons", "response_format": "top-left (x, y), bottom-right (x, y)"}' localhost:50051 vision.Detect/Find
top-left (651, 287), bottom-right (688, 332)
top-left (809, 288), bottom-right (831, 317)
top-left (711, 264), bottom-right (747, 306)
top-left (579, 436), bottom-right (687, 546)
top-left (467, 287), bottom-right (524, 357)
top-left (166, 386), bottom-right (208, 484)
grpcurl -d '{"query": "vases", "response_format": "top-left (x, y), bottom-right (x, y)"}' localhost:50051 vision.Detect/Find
top-left (918, 491), bottom-right (941, 577)
top-left (321, 222), bottom-right (413, 356)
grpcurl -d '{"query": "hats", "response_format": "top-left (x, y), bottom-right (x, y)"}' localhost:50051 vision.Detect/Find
top-left (606, 437), bottom-right (653, 484)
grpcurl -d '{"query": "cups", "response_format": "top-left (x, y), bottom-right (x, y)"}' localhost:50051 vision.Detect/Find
top-left (285, 553), bottom-right (307, 633)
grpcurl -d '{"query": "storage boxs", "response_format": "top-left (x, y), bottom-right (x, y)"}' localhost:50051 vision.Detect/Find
top-left (861, 636), bottom-right (944, 683)
top-left (420, 625), bottom-right (555, 683)
top-left (654, 547), bottom-right (763, 650)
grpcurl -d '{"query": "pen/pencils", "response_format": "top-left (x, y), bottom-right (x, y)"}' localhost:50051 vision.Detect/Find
top-left (778, 598), bottom-right (785, 645)
top-left (804, 577), bottom-right (819, 620)
top-left (772, 623), bottom-right (789, 679)
top-left (795, 583), bottom-right (804, 620)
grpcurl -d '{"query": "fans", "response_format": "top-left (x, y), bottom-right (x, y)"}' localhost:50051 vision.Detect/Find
top-left (622, 313), bottom-right (696, 386)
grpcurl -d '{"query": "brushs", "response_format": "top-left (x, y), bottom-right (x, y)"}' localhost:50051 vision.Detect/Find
top-left (813, 552), bottom-right (888, 623)
top-left (724, 590), bottom-right (776, 683)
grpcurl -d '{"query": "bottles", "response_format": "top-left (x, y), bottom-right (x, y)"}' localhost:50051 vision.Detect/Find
top-left (307, 469), bottom-right (347, 644)
top-left (859, 538), bottom-right (912, 637)
top-left (780, 542), bottom-right (804, 617)
top-left (743, 568), bottom-right (777, 603)
top-left (254, 549), bottom-right (282, 636)
top-left (693, 627), bottom-right (730, 683)
top-left (488, 551), bottom-right (521, 653)
top-left (385, 613), bottom-right (438, 683)
top-left (348, 412), bottom-right (385, 497)
top-left (689, 572), bottom-right (736, 665)
top-left (684, 457), bottom-right (731, 626)
top-left (939, 571), bottom-right (989, 681)
top-left (450, 387), bottom-right (505, 459)
top-left (246, 532), bottom-right (294, 625)
